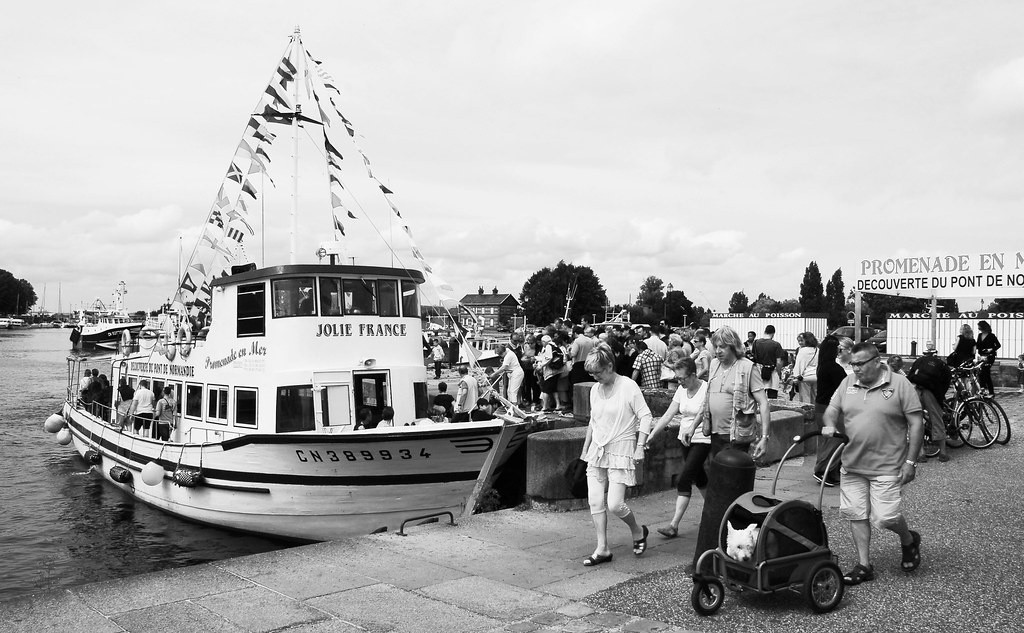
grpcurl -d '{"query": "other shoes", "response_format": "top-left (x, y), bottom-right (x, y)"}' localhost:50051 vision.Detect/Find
top-left (939, 454), bottom-right (949, 462)
top-left (518, 402), bottom-right (526, 410)
top-left (813, 471), bottom-right (840, 487)
top-left (917, 454), bottom-right (928, 463)
top-left (553, 409), bottom-right (562, 414)
top-left (541, 409), bottom-right (548, 413)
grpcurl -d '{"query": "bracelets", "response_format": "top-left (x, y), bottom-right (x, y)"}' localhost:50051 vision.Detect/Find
top-left (904, 459), bottom-right (918, 468)
top-left (759, 434), bottom-right (771, 440)
top-left (636, 443), bottom-right (644, 448)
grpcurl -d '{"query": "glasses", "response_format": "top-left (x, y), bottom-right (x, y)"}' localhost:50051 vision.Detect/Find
top-left (694, 340), bottom-right (700, 343)
top-left (674, 374), bottom-right (692, 382)
top-left (848, 356), bottom-right (875, 368)
top-left (588, 366), bottom-right (607, 377)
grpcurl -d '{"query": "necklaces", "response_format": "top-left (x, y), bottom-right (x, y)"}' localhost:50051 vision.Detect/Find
top-left (691, 384), bottom-right (700, 398)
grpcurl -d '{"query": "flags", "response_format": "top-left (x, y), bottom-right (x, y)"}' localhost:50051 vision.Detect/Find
top-left (153, 49), bottom-right (481, 369)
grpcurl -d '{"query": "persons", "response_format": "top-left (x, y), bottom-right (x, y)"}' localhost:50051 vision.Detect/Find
top-left (644, 357), bottom-right (712, 537)
top-left (976, 320), bottom-right (1002, 399)
top-left (952, 324), bottom-right (977, 391)
top-left (579, 339), bottom-right (653, 567)
top-left (79, 316), bottom-right (1024, 586)
top-left (822, 342), bottom-right (921, 585)
top-left (681, 324), bottom-right (771, 460)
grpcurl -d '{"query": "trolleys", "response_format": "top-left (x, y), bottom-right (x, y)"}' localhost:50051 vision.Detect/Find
top-left (689, 428), bottom-right (851, 617)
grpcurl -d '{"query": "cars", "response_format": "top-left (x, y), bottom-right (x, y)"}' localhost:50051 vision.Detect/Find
top-left (865, 329), bottom-right (886, 353)
top-left (829, 325), bottom-right (881, 343)
top-left (427, 323), bottom-right (485, 330)
top-left (496, 324), bottom-right (545, 334)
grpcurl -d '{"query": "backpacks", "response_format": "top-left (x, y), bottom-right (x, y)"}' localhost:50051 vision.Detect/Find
top-left (547, 344), bottom-right (564, 369)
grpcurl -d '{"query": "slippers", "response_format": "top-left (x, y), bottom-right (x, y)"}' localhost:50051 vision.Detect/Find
top-left (583, 550), bottom-right (612, 566)
top-left (633, 525), bottom-right (649, 555)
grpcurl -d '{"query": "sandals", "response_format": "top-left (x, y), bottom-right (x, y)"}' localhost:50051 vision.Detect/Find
top-left (901, 530), bottom-right (920, 572)
top-left (843, 562), bottom-right (875, 584)
top-left (658, 526), bottom-right (678, 536)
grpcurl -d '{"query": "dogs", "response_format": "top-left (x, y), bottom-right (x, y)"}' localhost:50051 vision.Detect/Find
top-left (725, 520), bottom-right (764, 561)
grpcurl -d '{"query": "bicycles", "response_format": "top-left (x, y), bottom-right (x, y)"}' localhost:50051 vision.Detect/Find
top-left (920, 360), bottom-right (1012, 458)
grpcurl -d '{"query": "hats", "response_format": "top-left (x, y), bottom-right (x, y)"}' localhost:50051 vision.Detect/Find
top-left (922, 340), bottom-right (937, 354)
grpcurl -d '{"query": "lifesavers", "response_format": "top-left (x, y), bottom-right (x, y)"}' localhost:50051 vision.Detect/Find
top-left (163, 317), bottom-right (176, 361)
top-left (120, 329), bottom-right (131, 356)
top-left (139, 326), bottom-right (158, 338)
top-left (177, 323), bottom-right (191, 356)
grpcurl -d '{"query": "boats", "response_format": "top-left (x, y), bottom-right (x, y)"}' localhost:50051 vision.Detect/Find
top-left (45, 250), bottom-right (559, 544)
top-left (423, 331), bottom-right (509, 368)
top-left (0, 318), bottom-right (76, 328)
top-left (70, 281), bottom-right (144, 342)
